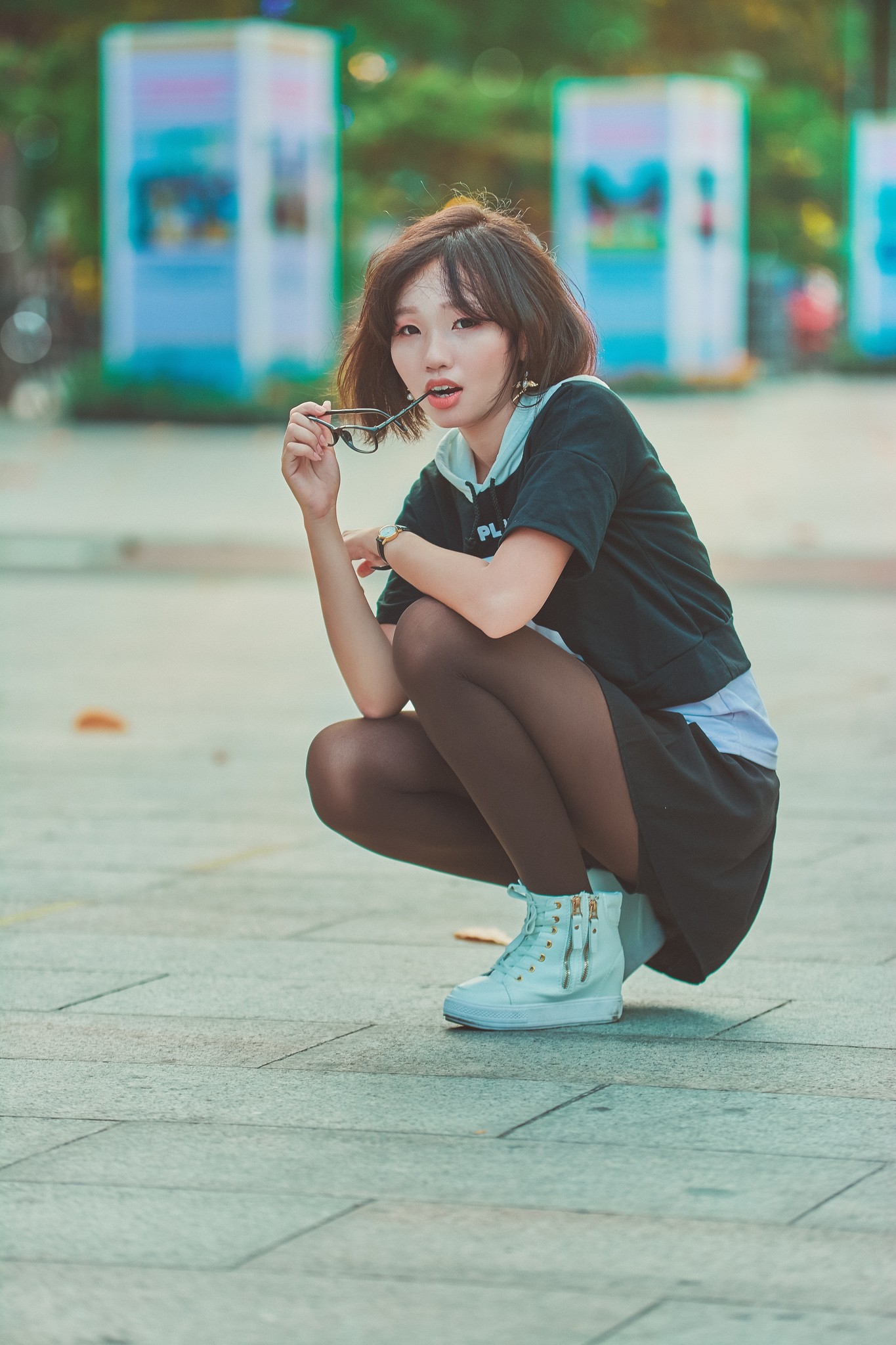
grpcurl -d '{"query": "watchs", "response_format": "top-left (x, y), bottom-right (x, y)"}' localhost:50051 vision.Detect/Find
top-left (369, 525), bottom-right (409, 570)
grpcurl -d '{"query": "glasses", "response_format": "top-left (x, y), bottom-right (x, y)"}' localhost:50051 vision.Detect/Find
top-left (307, 389), bottom-right (434, 454)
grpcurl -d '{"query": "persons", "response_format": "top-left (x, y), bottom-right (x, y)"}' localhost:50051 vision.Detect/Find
top-left (281, 208), bottom-right (778, 1033)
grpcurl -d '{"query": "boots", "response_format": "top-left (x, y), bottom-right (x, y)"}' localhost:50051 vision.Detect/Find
top-left (586, 866), bottom-right (667, 983)
top-left (442, 879), bottom-right (625, 1031)
